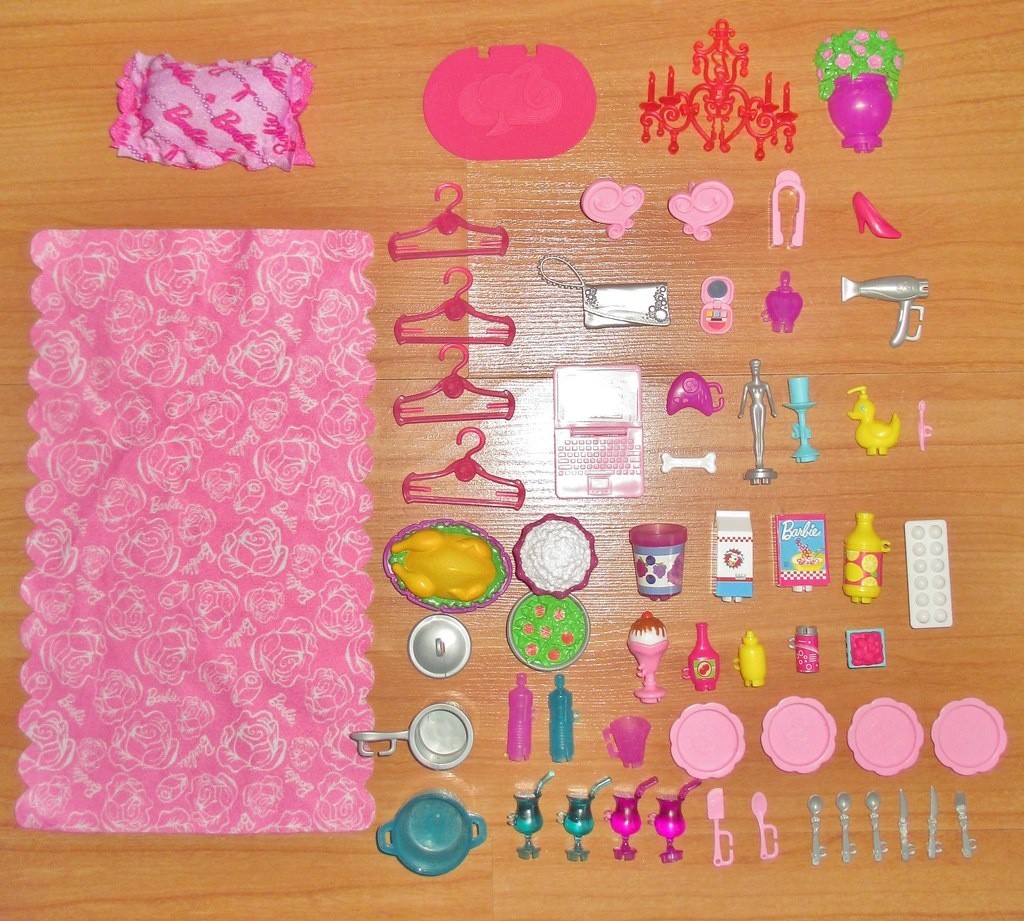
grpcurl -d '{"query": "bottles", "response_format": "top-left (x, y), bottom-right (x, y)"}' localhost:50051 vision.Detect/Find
top-left (682, 623), bottom-right (721, 691)
top-left (507, 674), bottom-right (538, 762)
top-left (843, 512), bottom-right (891, 603)
top-left (548, 674), bottom-right (581, 763)
top-left (733, 631), bottom-right (766, 687)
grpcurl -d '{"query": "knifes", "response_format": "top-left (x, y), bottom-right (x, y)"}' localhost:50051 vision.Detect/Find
top-left (706, 787), bottom-right (734, 867)
top-left (927, 786), bottom-right (942, 858)
top-left (898, 788), bottom-right (916, 861)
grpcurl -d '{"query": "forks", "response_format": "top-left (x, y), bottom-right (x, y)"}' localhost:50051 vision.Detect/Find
top-left (956, 792), bottom-right (977, 858)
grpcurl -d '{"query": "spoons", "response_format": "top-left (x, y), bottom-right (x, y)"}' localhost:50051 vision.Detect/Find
top-left (836, 792), bottom-right (857, 863)
top-left (808, 794), bottom-right (827, 865)
top-left (751, 792), bottom-right (779, 859)
top-left (865, 791), bottom-right (889, 861)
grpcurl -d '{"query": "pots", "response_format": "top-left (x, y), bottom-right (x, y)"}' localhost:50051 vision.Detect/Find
top-left (376, 792), bottom-right (487, 876)
top-left (351, 703), bottom-right (474, 771)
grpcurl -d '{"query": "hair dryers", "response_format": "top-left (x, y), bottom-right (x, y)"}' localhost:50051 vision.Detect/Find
top-left (840, 275), bottom-right (931, 350)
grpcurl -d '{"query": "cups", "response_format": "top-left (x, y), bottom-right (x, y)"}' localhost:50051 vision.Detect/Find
top-left (629, 523), bottom-right (686, 600)
top-left (603, 716), bottom-right (650, 768)
top-left (788, 626), bottom-right (819, 673)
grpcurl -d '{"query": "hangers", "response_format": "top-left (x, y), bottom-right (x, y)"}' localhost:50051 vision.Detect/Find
top-left (388, 182), bottom-right (510, 263)
top-left (402, 427), bottom-right (526, 510)
top-left (393, 267), bottom-right (516, 346)
top-left (393, 344), bottom-right (516, 427)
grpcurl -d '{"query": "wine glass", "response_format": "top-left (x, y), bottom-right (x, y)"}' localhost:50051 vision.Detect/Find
top-left (603, 776), bottom-right (658, 861)
top-left (556, 776), bottom-right (611, 861)
top-left (507, 770), bottom-right (555, 859)
top-left (648, 779), bottom-right (701, 863)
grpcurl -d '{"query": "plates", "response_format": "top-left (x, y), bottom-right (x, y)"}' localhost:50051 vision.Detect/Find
top-left (383, 519), bottom-right (513, 616)
top-left (931, 698), bottom-right (1008, 776)
top-left (512, 513), bottom-right (598, 598)
top-left (670, 702), bottom-right (745, 779)
top-left (847, 696), bottom-right (925, 776)
top-left (506, 592), bottom-right (590, 671)
top-left (760, 696), bottom-right (837, 773)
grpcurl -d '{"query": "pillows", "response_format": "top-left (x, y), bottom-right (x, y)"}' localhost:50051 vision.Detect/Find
top-left (108, 48), bottom-right (317, 171)
